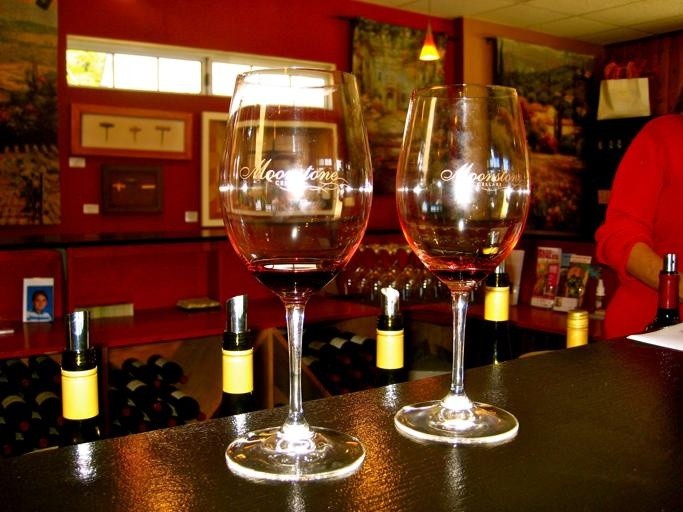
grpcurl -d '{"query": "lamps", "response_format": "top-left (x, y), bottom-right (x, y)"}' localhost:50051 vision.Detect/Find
top-left (418, 10), bottom-right (444, 63)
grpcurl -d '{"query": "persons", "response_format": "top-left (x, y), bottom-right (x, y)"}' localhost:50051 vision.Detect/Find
top-left (26, 290), bottom-right (54, 323)
top-left (594, 90), bottom-right (683, 338)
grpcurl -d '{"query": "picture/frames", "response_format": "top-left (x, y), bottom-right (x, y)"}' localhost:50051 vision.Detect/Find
top-left (99, 164), bottom-right (163, 216)
top-left (68, 157), bottom-right (85, 170)
top-left (184, 211), bottom-right (200, 223)
top-left (83, 203), bottom-right (99, 217)
top-left (70, 103), bottom-right (194, 159)
top-left (201, 112), bottom-right (344, 225)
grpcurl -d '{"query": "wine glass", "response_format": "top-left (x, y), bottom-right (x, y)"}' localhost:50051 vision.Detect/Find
top-left (344, 243), bottom-right (448, 304)
top-left (393, 84), bottom-right (531, 449)
top-left (217, 68), bottom-right (373, 489)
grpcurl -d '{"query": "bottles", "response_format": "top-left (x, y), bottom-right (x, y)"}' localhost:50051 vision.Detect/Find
top-left (594, 280), bottom-right (606, 315)
top-left (644, 252), bottom-right (683, 334)
top-left (479, 258), bottom-right (519, 368)
top-left (284, 330), bottom-right (375, 396)
top-left (210, 295), bottom-right (264, 420)
top-left (112, 353), bottom-right (207, 438)
top-left (375, 287), bottom-right (405, 388)
top-left (0, 354), bottom-right (61, 460)
top-left (58, 309), bottom-right (104, 448)
